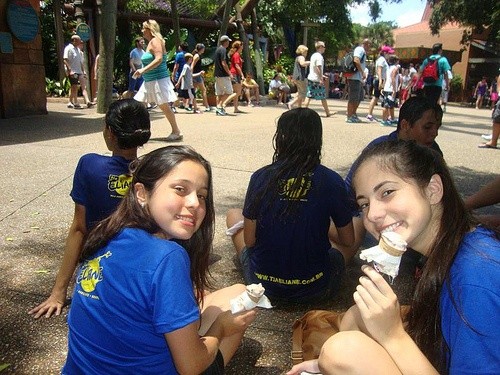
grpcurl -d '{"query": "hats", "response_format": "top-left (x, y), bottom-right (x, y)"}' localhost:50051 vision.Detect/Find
top-left (381, 46), bottom-right (395, 53)
top-left (219, 35), bottom-right (232, 42)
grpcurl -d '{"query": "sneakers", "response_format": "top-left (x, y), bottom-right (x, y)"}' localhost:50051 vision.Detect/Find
top-left (216, 107), bottom-right (227, 116)
top-left (389, 117), bottom-right (399, 126)
top-left (287, 102), bottom-right (293, 111)
top-left (67, 101), bottom-right (75, 108)
top-left (222, 104), bottom-right (228, 114)
top-left (74, 103), bottom-right (85, 109)
top-left (145, 100), bottom-right (212, 114)
top-left (87, 101), bottom-right (97, 109)
top-left (345, 112), bottom-right (362, 123)
top-left (381, 118), bottom-right (396, 127)
top-left (234, 109), bottom-right (245, 113)
top-left (367, 114), bottom-right (373, 121)
top-left (162, 131), bottom-right (183, 141)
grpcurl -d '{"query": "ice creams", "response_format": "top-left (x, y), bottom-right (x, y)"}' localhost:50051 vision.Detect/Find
top-left (243, 282), bottom-right (266, 310)
top-left (371, 231), bottom-right (408, 286)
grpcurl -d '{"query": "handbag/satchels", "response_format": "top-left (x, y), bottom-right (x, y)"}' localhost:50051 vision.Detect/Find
top-left (290, 305), bottom-right (413, 367)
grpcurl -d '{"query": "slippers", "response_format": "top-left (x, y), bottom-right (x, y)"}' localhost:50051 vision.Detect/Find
top-left (478, 142), bottom-right (498, 148)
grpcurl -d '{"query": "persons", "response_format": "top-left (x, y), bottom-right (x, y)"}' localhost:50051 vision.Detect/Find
top-left (93, 53), bottom-right (99, 81)
top-left (366, 46), bottom-right (396, 121)
top-left (27, 97), bottom-right (151, 319)
top-left (60, 144), bottom-right (259, 375)
top-left (342, 94), bottom-right (444, 269)
top-left (464, 176), bottom-right (500, 239)
top-left (172, 53), bottom-right (201, 114)
top-left (238, 71), bottom-right (261, 107)
top-left (477, 99), bottom-right (500, 148)
top-left (132, 19), bottom-right (183, 142)
top-left (226, 108), bottom-right (367, 306)
top-left (345, 38), bottom-right (372, 123)
top-left (171, 41), bottom-right (193, 109)
top-left (411, 43), bottom-right (451, 103)
top-left (63, 35), bottom-right (85, 109)
top-left (268, 71), bottom-right (289, 105)
top-left (286, 139), bottom-right (499, 375)
top-left (380, 55), bottom-right (401, 127)
top-left (214, 35), bottom-right (237, 116)
top-left (303, 40), bottom-right (338, 117)
top-left (223, 41), bottom-right (247, 114)
top-left (286, 45), bottom-right (311, 110)
top-left (324, 67), bottom-right (499, 109)
top-left (76, 39), bottom-right (97, 108)
top-left (122, 37), bottom-right (145, 100)
top-left (190, 43), bottom-right (214, 112)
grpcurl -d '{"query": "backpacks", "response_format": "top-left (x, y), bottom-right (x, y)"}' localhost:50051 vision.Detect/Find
top-left (421, 55), bottom-right (444, 83)
top-left (342, 48), bottom-right (365, 77)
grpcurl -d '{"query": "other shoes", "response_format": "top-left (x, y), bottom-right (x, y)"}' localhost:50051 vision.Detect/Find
top-left (327, 110), bottom-right (337, 117)
